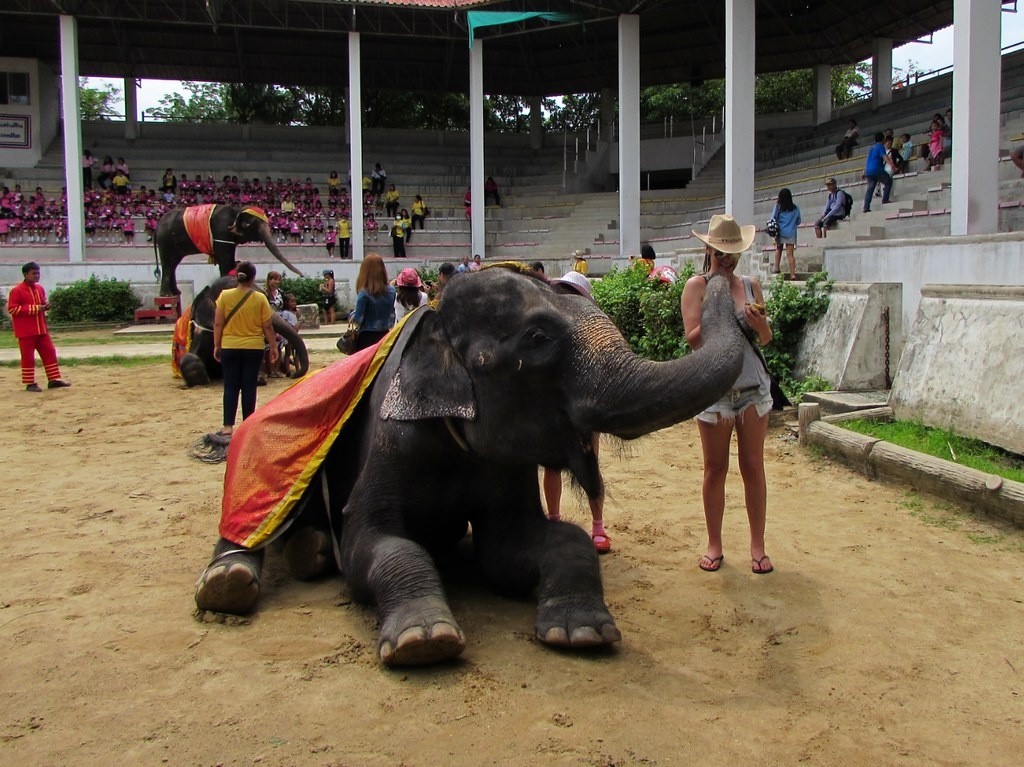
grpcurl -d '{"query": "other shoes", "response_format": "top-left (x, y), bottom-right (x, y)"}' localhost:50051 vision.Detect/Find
top-left (863, 209), bottom-right (871, 212)
top-left (48, 380), bottom-right (71, 388)
top-left (882, 199), bottom-right (892, 204)
top-left (268, 372), bottom-right (286, 378)
top-left (27, 383), bottom-right (42, 392)
top-left (592, 534), bottom-right (611, 553)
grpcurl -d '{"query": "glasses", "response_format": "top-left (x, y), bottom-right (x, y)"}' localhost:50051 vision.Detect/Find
top-left (711, 249), bottom-right (741, 261)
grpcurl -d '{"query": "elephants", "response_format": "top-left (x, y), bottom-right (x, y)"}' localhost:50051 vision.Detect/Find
top-left (154, 204), bottom-right (305, 297)
top-left (195, 261), bottom-right (745, 671)
top-left (172, 276), bottom-right (309, 388)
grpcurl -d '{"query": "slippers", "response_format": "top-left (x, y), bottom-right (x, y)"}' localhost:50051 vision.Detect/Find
top-left (752, 556), bottom-right (773, 573)
top-left (699, 554), bottom-right (723, 571)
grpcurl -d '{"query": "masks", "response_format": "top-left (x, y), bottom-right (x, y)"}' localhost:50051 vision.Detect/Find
top-left (397, 216), bottom-right (401, 220)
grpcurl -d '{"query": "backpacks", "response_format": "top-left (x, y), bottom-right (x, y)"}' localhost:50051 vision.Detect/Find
top-left (829, 189), bottom-right (853, 218)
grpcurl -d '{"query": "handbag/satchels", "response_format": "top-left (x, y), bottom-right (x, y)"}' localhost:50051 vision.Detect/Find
top-left (765, 204), bottom-right (779, 237)
top-left (337, 316), bottom-right (361, 355)
top-left (420, 201), bottom-right (430, 215)
top-left (391, 226), bottom-right (397, 237)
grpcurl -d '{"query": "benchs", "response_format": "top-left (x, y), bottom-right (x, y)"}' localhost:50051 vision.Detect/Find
top-left (0, 50), bottom-right (1024, 268)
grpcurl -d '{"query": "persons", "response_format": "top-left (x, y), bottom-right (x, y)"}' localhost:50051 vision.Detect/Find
top-left (628, 244), bottom-right (656, 276)
top-left (213, 261), bottom-right (280, 436)
top-left (320, 269), bottom-right (336, 324)
top-left (862, 108), bottom-right (953, 213)
top-left (0, 149), bottom-right (504, 259)
top-left (456, 257), bottom-right (472, 272)
top-left (814, 177), bottom-right (846, 238)
top-left (391, 268), bottom-right (428, 326)
top-left (770, 187), bottom-right (802, 282)
top-left (423, 263), bottom-right (459, 302)
top-left (470, 254), bottom-right (483, 271)
top-left (681, 213), bottom-right (775, 574)
top-left (260, 270), bottom-right (301, 373)
top-left (532, 262), bottom-right (551, 281)
top-left (541, 272), bottom-right (612, 553)
top-left (6, 262), bottom-right (71, 391)
top-left (571, 250), bottom-right (589, 276)
top-left (347, 252), bottom-right (396, 353)
top-left (1010, 144), bottom-right (1024, 178)
top-left (835, 120), bottom-right (863, 160)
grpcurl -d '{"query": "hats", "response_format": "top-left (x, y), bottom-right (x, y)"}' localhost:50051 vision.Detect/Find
top-left (397, 267), bottom-right (422, 287)
top-left (322, 269), bottom-right (330, 275)
top-left (570, 250), bottom-right (584, 259)
top-left (552, 271), bottom-right (599, 307)
top-left (825, 178), bottom-right (835, 185)
top-left (692, 213), bottom-right (755, 254)
